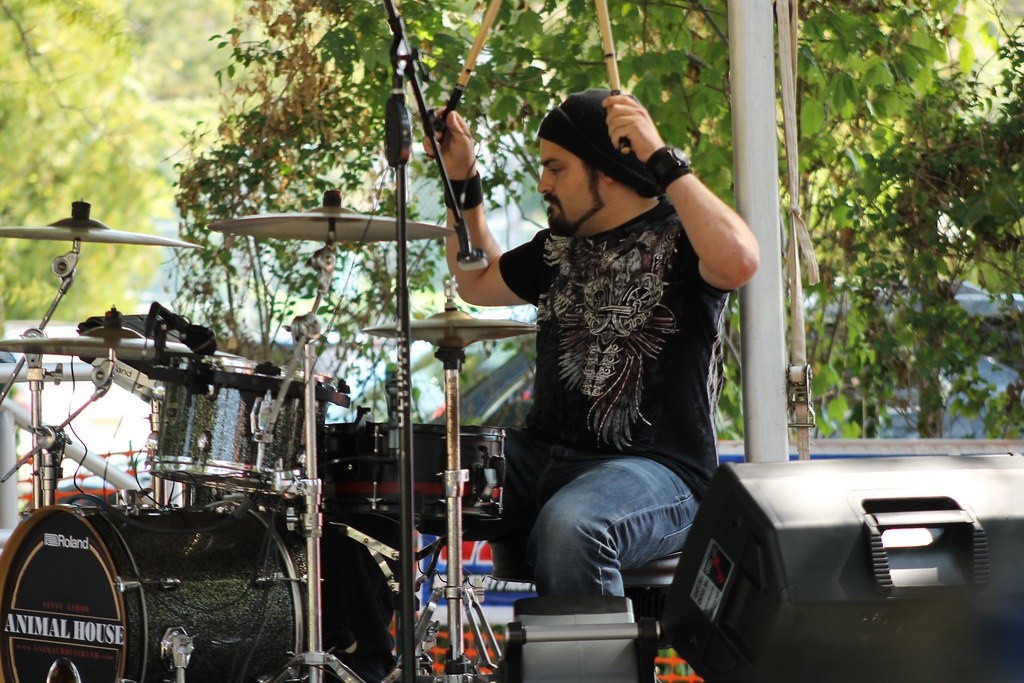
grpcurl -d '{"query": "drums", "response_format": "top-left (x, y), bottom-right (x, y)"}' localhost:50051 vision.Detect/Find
top-left (315, 418), bottom-right (511, 524)
top-left (149, 351), bottom-right (348, 501)
top-left (1, 493), bottom-right (323, 682)
top-left (317, 506), bottom-right (407, 683)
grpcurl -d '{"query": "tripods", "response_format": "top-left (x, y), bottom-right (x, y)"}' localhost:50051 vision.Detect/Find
top-left (259, 224), bottom-right (501, 682)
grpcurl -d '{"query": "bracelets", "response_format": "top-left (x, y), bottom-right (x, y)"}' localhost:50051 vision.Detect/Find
top-left (645, 146), bottom-right (693, 193)
top-left (444, 170), bottom-right (483, 210)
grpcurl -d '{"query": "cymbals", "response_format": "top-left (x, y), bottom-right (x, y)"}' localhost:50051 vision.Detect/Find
top-left (361, 311), bottom-right (543, 350)
top-left (206, 205), bottom-right (458, 246)
top-left (124, 357), bottom-right (353, 410)
top-left (0, 219), bottom-right (206, 251)
top-left (0, 334), bottom-right (253, 362)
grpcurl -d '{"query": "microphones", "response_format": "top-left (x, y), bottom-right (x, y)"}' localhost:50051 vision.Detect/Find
top-left (151, 302), bottom-right (218, 356)
top-left (454, 220), bottom-right (489, 270)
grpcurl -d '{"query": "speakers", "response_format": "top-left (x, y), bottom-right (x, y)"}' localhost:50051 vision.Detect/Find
top-left (657, 455), bottom-right (1023, 683)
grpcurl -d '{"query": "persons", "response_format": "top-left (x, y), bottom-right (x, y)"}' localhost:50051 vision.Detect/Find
top-left (423, 90), bottom-right (759, 597)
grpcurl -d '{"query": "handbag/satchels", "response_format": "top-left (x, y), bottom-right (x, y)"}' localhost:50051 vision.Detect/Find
top-left (321, 512), bottom-right (420, 683)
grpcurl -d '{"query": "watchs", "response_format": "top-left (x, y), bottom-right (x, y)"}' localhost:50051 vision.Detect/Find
top-left (648, 147), bottom-right (690, 185)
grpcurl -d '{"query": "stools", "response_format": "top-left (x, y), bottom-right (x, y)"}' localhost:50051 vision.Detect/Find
top-left (492, 539), bottom-right (685, 683)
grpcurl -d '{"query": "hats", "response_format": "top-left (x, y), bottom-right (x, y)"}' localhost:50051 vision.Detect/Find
top-left (538, 89), bottom-right (661, 195)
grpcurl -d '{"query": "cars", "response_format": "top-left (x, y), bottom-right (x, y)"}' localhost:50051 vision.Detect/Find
top-left (429, 268), bottom-right (1024, 448)
top-left (1, 320), bottom-right (186, 521)
